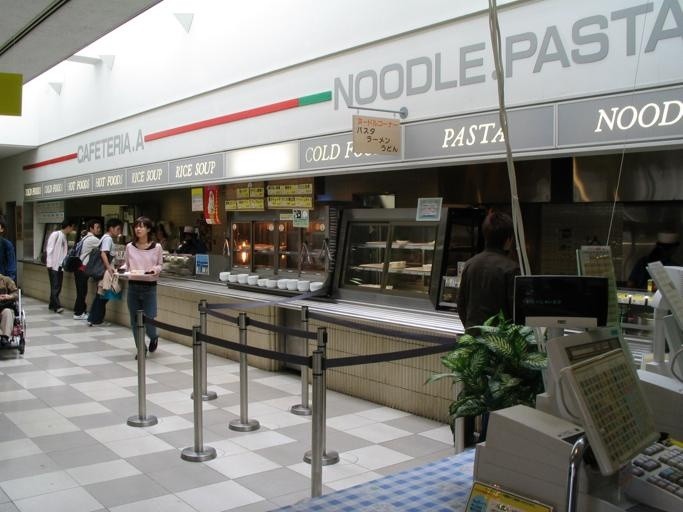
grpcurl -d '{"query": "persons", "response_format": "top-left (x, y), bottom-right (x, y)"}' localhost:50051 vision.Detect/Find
top-left (625, 222), bottom-right (682, 289)
top-left (0, 272), bottom-right (19, 348)
top-left (85, 218), bottom-right (121, 327)
top-left (0, 217), bottom-right (16, 292)
top-left (71, 221), bottom-right (101, 320)
top-left (455, 206), bottom-right (523, 446)
top-left (113, 215), bottom-right (163, 360)
top-left (42, 218), bottom-right (76, 314)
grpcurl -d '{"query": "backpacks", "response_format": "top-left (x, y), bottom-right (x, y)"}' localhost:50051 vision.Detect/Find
top-left (60, 233), bottom-right (95, 273)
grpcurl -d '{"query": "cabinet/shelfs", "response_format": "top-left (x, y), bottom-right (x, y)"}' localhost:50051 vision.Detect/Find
top-left (333, 207), bottom-right (485, 313)
top-left (37, 215), bottom-right (104, 268)
top-left (228, 214), bottom-right (327, 277)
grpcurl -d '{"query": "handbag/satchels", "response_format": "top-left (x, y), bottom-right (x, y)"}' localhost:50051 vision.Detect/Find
top-left (41, 231), bottom-right (59, 265)
top-left (82, 235), bottom-right (115, 281)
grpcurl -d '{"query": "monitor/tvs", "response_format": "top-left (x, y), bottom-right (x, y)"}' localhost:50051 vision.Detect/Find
top-left (646, 261), bottom-right (683, 361)
top-left (560, 348), bottom-right (661, 476)
top-left (576, 246), bottom-right (619, 325)
top-left (545, 326), bottom-right (654, 419)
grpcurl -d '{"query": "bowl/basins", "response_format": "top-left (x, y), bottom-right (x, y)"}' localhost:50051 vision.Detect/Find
top-left (218, 272), bottom-right (323, 291)
top-left (380, 194), bottom-right (395, 209)
top-left (116, 268), bottom-right (126, 274)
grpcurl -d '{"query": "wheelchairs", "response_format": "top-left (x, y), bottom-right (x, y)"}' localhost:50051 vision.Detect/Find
top-left (0, 282), bottom-right (27, 357)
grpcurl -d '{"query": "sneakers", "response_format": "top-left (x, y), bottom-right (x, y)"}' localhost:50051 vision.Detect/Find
top-left (72, 311), bottom-right (112, 328)
top-left (134, 336), bottom-right (158, 361)
top-left (49, 304), bottom-right (64, 314)
top-left (0, 336), bottom-right (10, 350)
